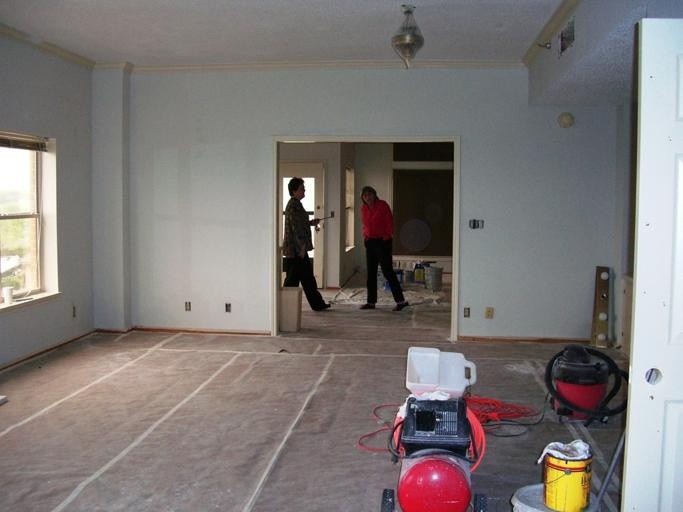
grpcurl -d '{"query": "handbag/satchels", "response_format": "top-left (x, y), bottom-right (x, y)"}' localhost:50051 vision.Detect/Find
top-left (283, 257), bottom-right (312, 272)
top-left (365, 238), bottom-right (381, 248)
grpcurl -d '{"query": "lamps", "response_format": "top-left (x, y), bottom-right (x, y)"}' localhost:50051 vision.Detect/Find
top-left (389, 4), bottom-right (423, 71)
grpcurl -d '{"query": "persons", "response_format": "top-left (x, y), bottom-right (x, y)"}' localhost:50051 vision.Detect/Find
top-left (281, 176), bottom-right (332, 311)
top-left (358, 186), bottom-right (409, 312)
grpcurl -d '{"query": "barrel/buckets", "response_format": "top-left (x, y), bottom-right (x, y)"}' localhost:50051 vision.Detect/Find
top-left (541, 456), bottom-right (593, 512)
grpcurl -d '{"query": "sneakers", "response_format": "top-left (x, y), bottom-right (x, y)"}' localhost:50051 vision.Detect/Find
top-left (392, 302), bottom-right (408, 311)
top-left (360, 304), bottom-right (375, 308)
top-left (312, 304), bottom-right (330, 310)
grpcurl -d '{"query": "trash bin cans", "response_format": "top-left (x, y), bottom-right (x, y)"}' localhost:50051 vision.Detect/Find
top-left (385, 269), bottom-right (403, 293)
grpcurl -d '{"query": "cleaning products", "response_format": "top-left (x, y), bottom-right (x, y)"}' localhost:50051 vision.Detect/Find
top-left (413, 259), bottom-right (425, 283)
